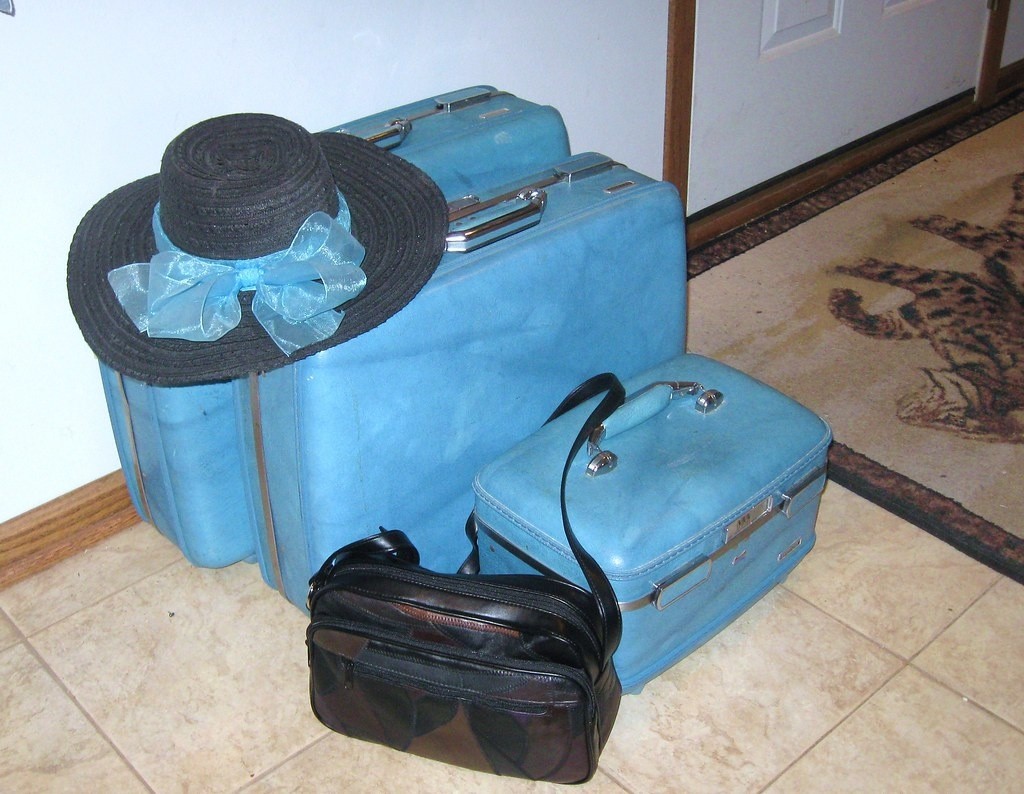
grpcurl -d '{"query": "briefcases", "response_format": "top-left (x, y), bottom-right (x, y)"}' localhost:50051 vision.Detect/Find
top-left (472, 352), bottom-right (832, 695)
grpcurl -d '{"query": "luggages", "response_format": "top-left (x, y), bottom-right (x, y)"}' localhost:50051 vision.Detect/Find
top-left (98, 85), bottom-right (688, 618)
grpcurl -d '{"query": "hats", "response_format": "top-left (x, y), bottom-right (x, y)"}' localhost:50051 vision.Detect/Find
top-left (66, 113), bottom-right (451, 385)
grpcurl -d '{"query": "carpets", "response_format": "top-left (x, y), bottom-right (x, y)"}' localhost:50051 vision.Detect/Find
top-left (688, 82), bottom-right (1024, 586)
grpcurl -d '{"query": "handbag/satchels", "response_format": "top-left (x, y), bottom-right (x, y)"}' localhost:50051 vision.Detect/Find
top-left (306, 527), bottom-right (624, 786)
